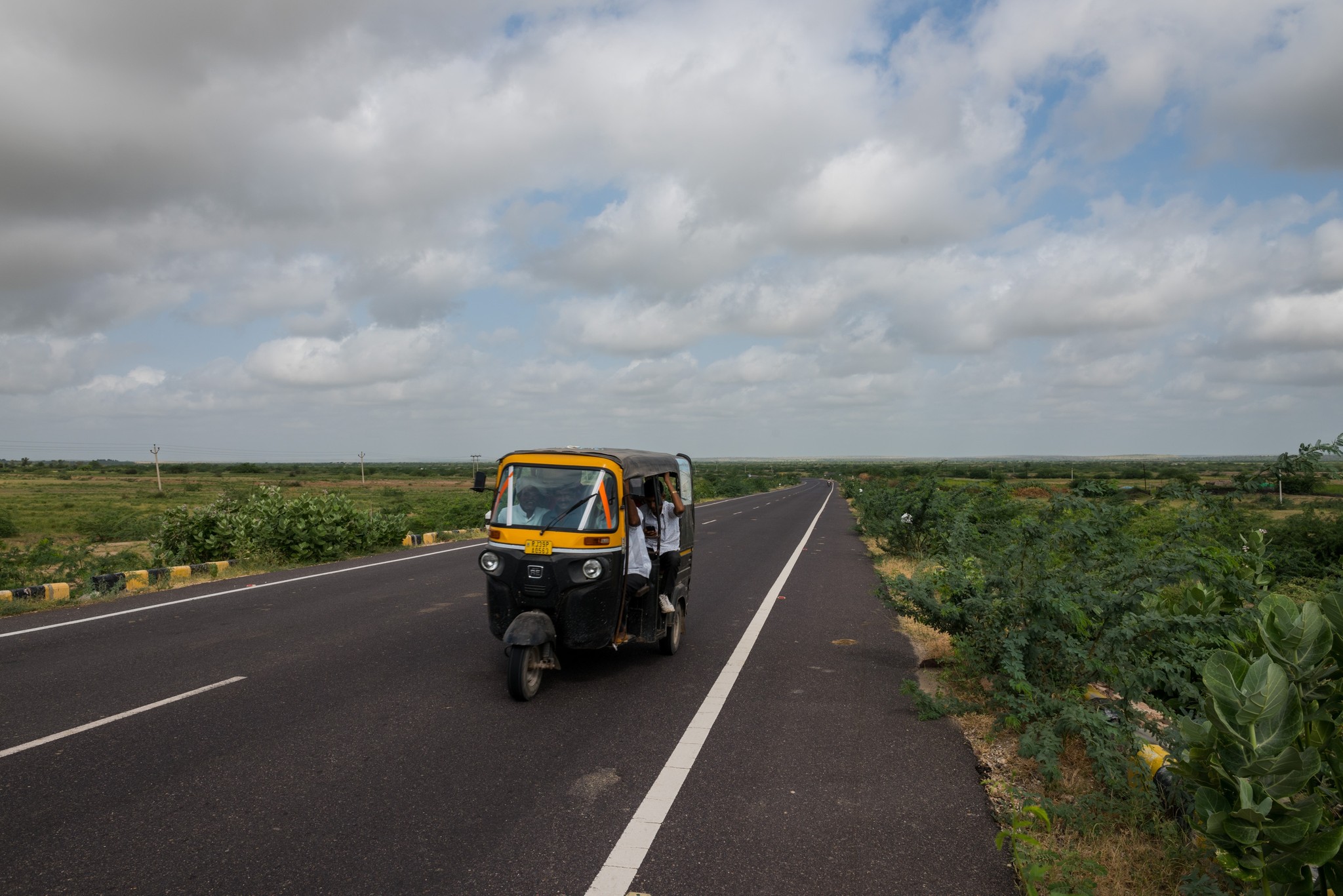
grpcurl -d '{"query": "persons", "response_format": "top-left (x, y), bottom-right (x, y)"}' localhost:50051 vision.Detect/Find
top-left (639, 472), bottom-right (685, 614)
top-left (497, 477), bottom-right (550, 526)
top-left (827, 478), bottom-right (831, 484)
top-left (540, 482), bottom-right (595, 529)
top-left (592, 479), bottom-right (653, 645)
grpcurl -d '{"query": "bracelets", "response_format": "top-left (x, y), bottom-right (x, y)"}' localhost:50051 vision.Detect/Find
top-left (670, 491), bottom-right (678, 496)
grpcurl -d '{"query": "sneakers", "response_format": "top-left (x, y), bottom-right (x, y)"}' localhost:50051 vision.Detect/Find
top-left (658, 594), bottom-right (675, 613)
top-left (636, 584), bottom-right (649, 597)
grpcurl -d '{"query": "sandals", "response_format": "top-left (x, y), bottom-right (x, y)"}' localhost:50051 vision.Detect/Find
top-left (611, 633), bottom-right (628, 644)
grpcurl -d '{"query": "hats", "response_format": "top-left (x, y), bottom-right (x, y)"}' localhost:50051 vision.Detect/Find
top-left (514, 475), bottom-right (547, 498)
top-left (552, 480), bottom-right (572, 497)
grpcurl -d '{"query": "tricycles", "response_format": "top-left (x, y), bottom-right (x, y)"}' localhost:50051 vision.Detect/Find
top-left (470, 444), bottom-right (695, 702)
